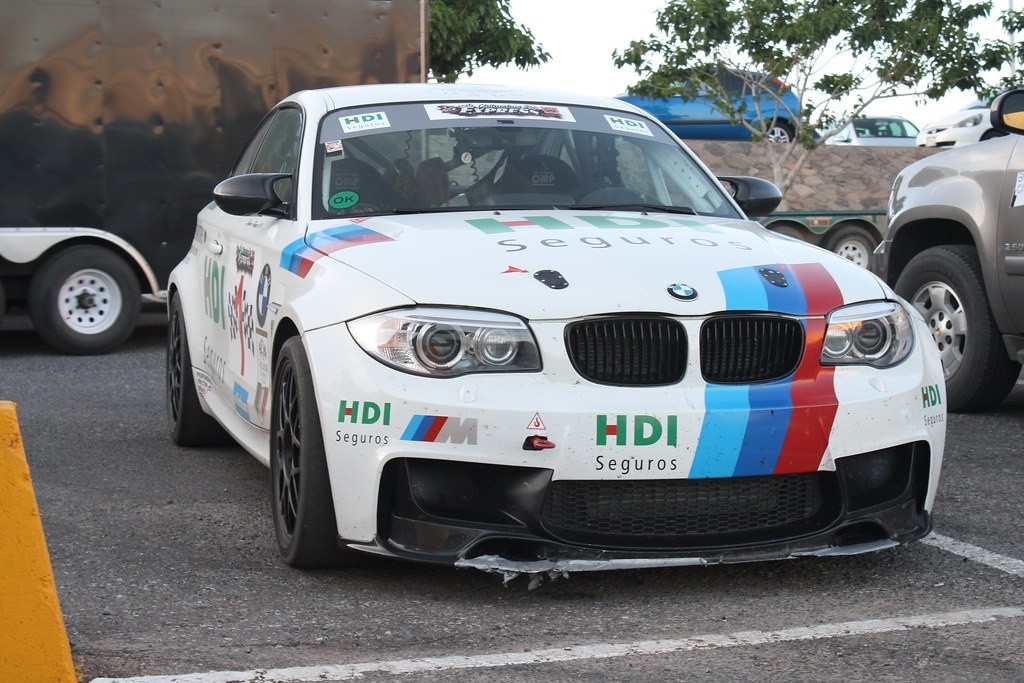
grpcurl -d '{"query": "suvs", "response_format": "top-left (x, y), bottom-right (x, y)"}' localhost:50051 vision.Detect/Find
top-left (816, 115), bottom-right (922, 147)
top-left (881, 86), bottom-right (1024, 416)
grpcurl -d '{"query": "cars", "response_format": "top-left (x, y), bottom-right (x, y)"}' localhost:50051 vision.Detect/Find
top-left (168, 82), bottom-right (950, 577)
top-left (606, 65), bottom-right (806, 144)
top-left (914, 92), bottom-right (1009, 149)
top-left (0, 226), bottom-right (160, 352)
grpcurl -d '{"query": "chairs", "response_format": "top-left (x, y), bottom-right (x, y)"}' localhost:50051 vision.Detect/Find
top-left (331, 158), bottom-right (395, 209)
top-left (501, 154), bottom-right (589, 200)
